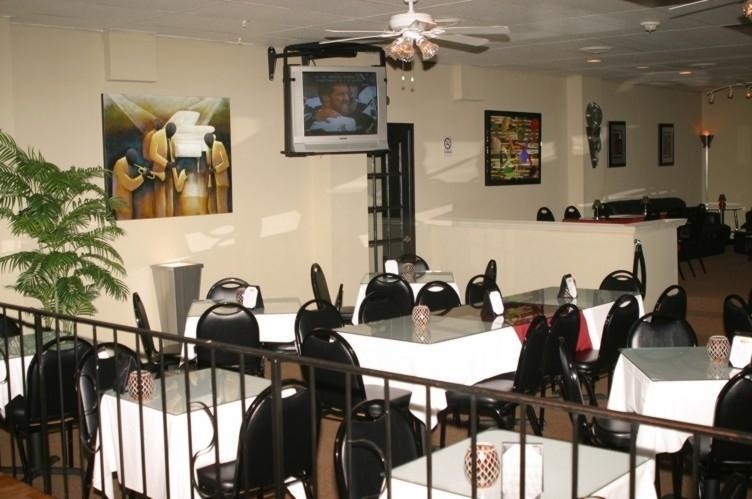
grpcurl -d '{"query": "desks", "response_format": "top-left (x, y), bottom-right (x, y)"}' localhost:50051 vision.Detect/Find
top-left (706, 207), bottom-right (741, 230)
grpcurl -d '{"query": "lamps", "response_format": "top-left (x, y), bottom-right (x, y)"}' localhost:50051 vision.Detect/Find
top-left (699, 130), bottom-right (715, 202)
top-left (743, 1), bottom-right (752, 19)
top-left (383, 0), bottom-right (439, 82)
top-left (706, 82), bottom-right (751, 104)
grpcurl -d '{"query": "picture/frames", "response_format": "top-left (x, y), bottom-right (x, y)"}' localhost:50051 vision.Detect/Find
top-left (658, 123), bottom-right (675, 165)
top-left (608, 120), bottom-right (629, 167)
top-left (484, 109), bottom-right (542, 186)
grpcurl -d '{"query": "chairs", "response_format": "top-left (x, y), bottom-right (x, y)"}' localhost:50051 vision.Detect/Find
top-left (536, 206), bottom-right (707, 279)
top-left (0, 245), bottom-right (752, 499)
top-left (736, 210), bottom-right (752, 255)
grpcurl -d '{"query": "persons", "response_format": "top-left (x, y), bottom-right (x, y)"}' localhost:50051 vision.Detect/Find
top-left (111, 119), bottom-right (230, 220)
top-left (304, 79), bottom-right (377, 133)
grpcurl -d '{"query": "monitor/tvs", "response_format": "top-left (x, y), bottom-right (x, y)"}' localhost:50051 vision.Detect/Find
top-left (289, 64), bottom-right (391, 153)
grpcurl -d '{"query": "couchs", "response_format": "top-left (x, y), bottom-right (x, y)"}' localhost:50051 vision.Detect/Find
top-left (602, 197), bottom-right (732, 260)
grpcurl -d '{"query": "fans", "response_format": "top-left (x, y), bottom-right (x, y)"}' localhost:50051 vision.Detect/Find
top-left (319, 0), bottom-right (511, 83)
top-left (668, 0), bottom-right (752, 19)
